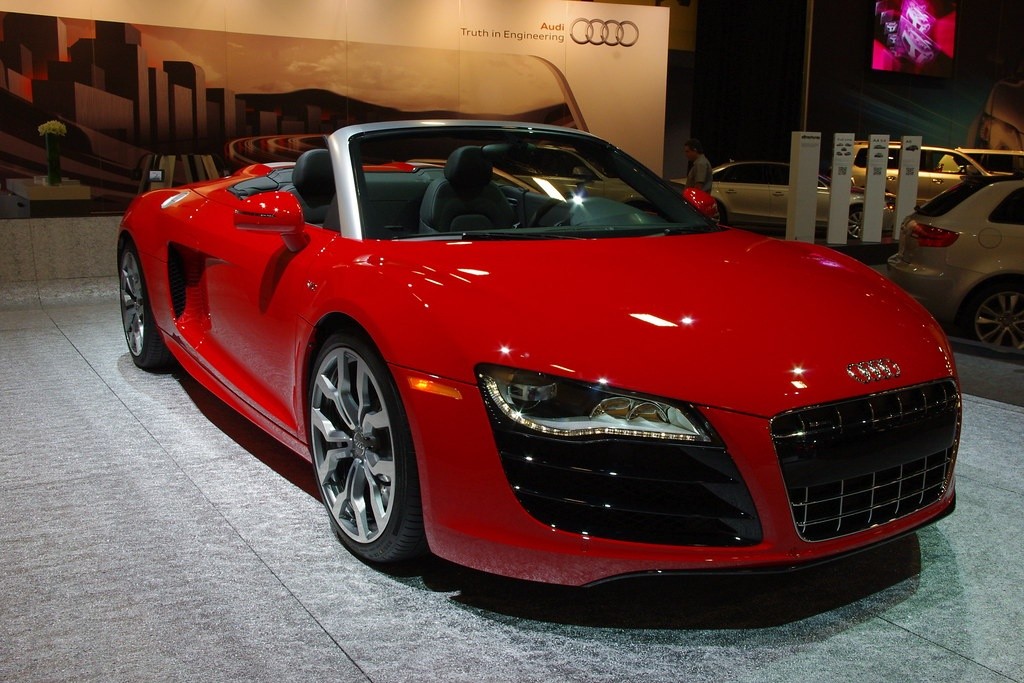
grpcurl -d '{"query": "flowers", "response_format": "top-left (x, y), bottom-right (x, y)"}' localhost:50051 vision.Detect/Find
top-left (37, 119), bottom-right (67, 138)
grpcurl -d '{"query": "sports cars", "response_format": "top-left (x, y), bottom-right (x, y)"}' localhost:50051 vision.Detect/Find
top-left (114, 116), bottom-right (969, 592)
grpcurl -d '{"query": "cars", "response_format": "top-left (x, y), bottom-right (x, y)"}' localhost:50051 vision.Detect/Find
top-left (669, 161), bottom-right (904, 239)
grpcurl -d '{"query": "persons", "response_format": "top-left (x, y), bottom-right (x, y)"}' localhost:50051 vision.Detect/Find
top-left (683, 138), bottom-right (712, 193)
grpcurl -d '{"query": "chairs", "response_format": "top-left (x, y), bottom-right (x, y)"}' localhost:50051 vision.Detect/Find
top-left (280, 148), bottom-right (394, 241)
top-left (418, 144), bottom-right (523, 235)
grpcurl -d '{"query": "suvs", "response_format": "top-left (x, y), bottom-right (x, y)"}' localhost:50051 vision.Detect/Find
top-left (833, 138), bottom-right (990, 206)
top-left (937, 145), bottom-right (1024, 181)
top-left (884, 175), bottom-right (1024, 352)
top-left (497, 147), bottom-right (655, 215)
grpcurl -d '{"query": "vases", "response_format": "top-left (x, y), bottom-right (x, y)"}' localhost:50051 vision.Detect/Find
top-left (45, 135), bottom-right (62, 185)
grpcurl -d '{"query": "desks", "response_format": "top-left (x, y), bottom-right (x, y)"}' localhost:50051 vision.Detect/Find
top-left (6, 176), bottom-right (92, 217)
